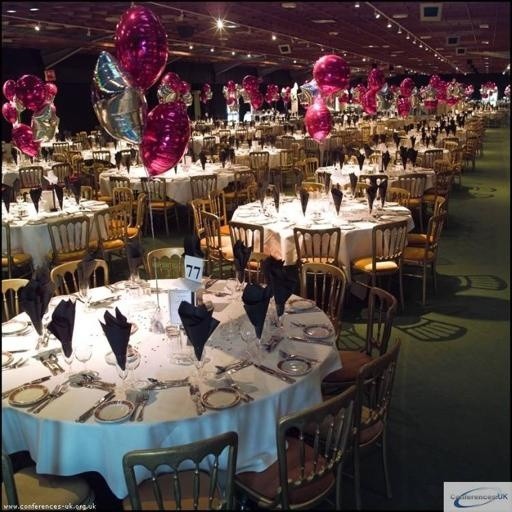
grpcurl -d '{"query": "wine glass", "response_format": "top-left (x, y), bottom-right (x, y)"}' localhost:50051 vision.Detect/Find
top-left (34, 270), bottom-right (287, 379)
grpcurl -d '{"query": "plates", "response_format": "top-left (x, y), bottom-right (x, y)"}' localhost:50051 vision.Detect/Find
top-left (337, 223), bottom-right (356, 230)
top-left (0, 294), bottom-right (336, 424)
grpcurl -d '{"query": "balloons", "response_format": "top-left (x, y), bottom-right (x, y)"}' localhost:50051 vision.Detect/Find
top-left (92, 4), bottom-right (193, 178)
top-left (1, 75), bottom-right (58, 157)
top-left (199, 55), bottom-right (497, 144)
